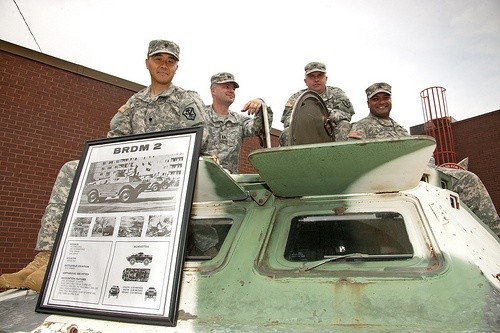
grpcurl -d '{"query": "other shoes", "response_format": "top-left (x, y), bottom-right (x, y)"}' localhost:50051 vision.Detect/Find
top-left (1, 251), bottom-right (50, 290)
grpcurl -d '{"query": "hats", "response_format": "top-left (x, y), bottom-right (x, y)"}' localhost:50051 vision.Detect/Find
top-left (304, 62), bottom-right (327, 74)
top-left (365, 82), bottom-right (391, 98)
top-left (148, 39), bottom-right (178, 59)
top-left (212, 72), bottom-right (240, 88)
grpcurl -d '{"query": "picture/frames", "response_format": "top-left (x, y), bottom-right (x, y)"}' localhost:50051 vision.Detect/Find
top-left (35, 125), bottom-right (204, 327)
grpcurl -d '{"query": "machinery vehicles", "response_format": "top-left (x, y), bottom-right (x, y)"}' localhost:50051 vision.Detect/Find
top-left (0, 89), bottom-right (500, 333)
top-left (85, 171), bottom-right (175, 204)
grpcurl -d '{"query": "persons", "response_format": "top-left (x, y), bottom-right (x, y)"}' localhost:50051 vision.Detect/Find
top-left (190, 72), bottom-right (273, 259)
top-left (0, 39), bottom-right (209, 290)
top-left (347, 82), bottom-right (500, 239)
top-left (278, 62), bottom-right (355, 147)
